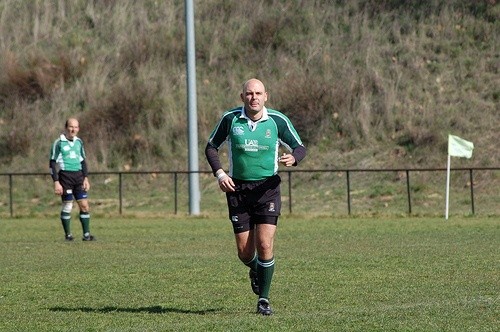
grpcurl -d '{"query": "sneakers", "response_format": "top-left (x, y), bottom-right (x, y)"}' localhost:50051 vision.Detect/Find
top-left (249, 269), bottom-right (259, 295)
top-left (257, 298), bottom-right (271, 315)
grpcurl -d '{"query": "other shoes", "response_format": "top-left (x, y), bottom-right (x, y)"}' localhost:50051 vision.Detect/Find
top-left (82, 232), bottom-right (96, 241)
top-left (65, 233), bottom-right (74, 241)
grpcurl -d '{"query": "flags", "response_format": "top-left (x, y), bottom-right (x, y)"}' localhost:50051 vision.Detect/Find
top-left (448, 133), bottom-right (475, 159)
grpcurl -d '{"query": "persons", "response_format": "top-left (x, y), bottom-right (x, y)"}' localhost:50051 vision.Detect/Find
top-left (47, 118), bottom-right (96, 240)
top-left (204, 78), bottom-right (307, 316)
